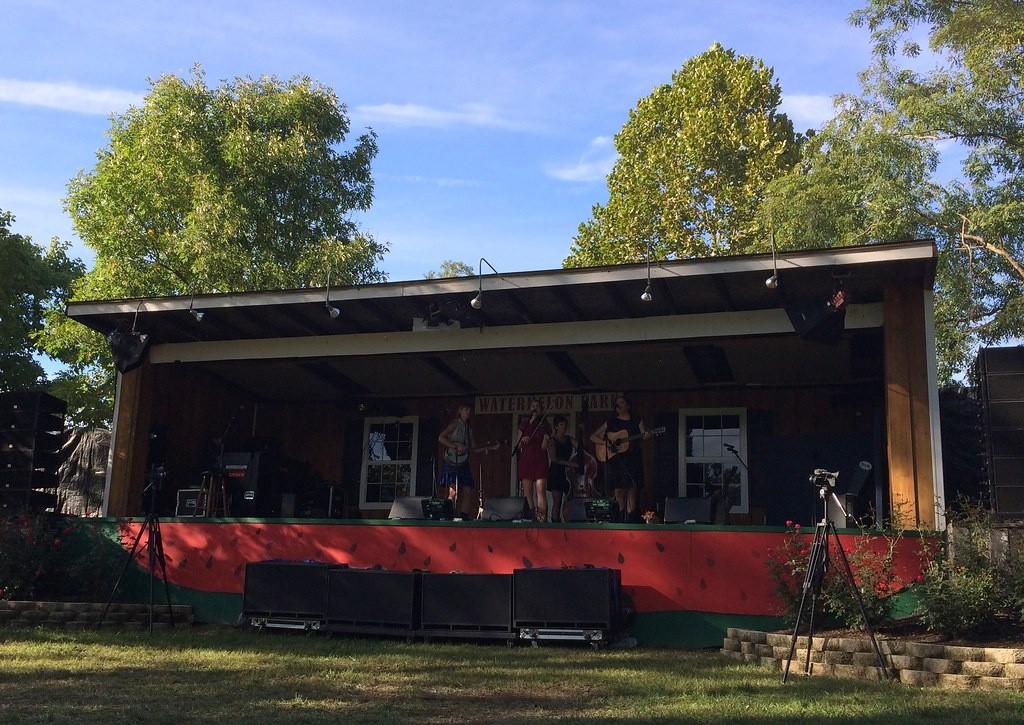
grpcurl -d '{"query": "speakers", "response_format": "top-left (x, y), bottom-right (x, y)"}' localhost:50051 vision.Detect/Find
top-left (242, 558), bottom-right (621, 633)
top-left (0, 391), bottom-right (67, 509)
top-left (482, 496), bottom-right (532, 522)
top-left (388, 495), bottom-right (431, 519)
top-left (570, 497), bottom-right (621, 523)
top-left (975, 345), bottom-right (1024, 517)
top-left (664, 495), bottom-right (718, 524)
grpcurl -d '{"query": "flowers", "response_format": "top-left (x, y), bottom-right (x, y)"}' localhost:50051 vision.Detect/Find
top-left (642, 511), bottom-right (654, 520)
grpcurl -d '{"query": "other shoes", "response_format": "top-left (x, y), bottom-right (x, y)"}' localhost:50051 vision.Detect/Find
top-left (552, 517), bottom-right (563, 523)
top-left (529, 510), bottom-right (548, 523)
top-left (456, 512), bottom-right (470, 520)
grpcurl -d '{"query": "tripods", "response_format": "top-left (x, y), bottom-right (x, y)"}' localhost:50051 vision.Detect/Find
top-left (782, 485), bottom-right (890, 686)
top-left (97, 482), bottom-right (176, 636)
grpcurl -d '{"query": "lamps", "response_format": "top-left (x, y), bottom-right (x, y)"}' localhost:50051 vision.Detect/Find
top-left (470, 258), bottom-right (504, 308)
top-left (765, 232), bottom-right (779, 288)
top-left (189, 279), bottom-right (228, 321)
top-left (325, 270), bottom-right (360, 319)
top-left (640, 246), bottom-right (661, 301)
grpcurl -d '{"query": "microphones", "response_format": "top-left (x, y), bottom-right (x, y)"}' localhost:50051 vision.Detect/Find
top-left (723, 443), bottom-right (734, 448)
top-left (616, 404), bottom-right (619, 407)
top-left (533, 409), bottom-right (536, 412)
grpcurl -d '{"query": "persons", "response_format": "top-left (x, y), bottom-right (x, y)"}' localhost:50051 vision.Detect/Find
top-left (439, 404), bottom-right (501, 518)
top-left (590, 396), bottom-right (652, 523)
top-left (516, 399), bottom-right (553, 522)
top-left (546, 415), bottom-right (579, 522)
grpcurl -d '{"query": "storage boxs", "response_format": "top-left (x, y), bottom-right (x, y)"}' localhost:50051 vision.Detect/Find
top-left (175, 487), bottom-right (207, 518)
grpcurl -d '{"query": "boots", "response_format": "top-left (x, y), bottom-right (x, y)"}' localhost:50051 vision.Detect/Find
top-left (615, 513), bottom-right (638, 523)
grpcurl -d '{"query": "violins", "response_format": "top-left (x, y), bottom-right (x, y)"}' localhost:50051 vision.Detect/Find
top-left (533, 416), bottom-right (553, 440)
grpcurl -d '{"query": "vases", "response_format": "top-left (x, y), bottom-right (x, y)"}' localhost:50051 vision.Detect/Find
top-left (646, 519), bottom-right (654, 524)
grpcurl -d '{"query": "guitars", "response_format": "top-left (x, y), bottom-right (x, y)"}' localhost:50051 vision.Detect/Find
top-left (444, 439), bottom-right (507, 467)
top-left (596, 424), bottom-right (672, 464)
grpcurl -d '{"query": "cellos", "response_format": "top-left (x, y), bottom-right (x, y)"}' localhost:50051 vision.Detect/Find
top-left (562, 400), bottom-right (602, 521)
